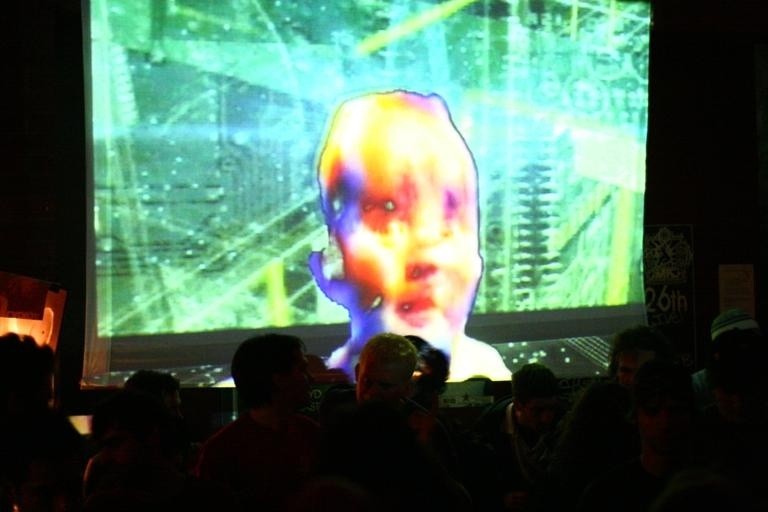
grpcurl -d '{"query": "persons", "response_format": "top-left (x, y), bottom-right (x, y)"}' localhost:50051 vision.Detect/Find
top-left (0, 304), bottom-right (767, 511)
top-left (303, 89), bottom-right (524, 385)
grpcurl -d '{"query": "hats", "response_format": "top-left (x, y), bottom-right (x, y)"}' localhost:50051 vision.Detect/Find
top-left (711, 307), bottom-right (760, 339)
